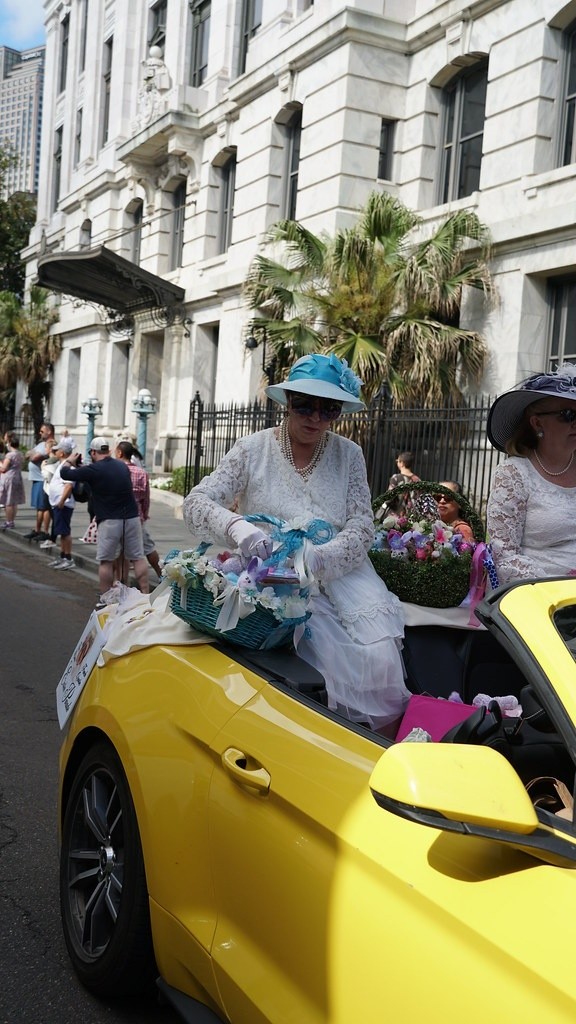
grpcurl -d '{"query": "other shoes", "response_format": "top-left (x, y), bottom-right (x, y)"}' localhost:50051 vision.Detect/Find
top-left (48, 555), bottom-right (68, 567)
top-left (54, 557), bottom-right (76, 571)
top-left (23, 529), bottom-right (40, 538)
top-left (0, 521), bottom-right (15, 530)
top-left (39, 540), bottom-right (57, 549)
top-left (33, 531), bottom-right (51, 541)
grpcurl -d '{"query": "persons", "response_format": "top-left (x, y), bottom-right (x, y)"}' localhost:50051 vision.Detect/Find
top-left (101, 353), bottom-right (413, 732)
top-left (117, 432), bottom-right (143, 468)
top-left (116, 441), bottom-right (165, 588)
top-left (376, 451), bottom-right (473, 540)
top-left (60, 437), bottom-right (149, 607)
top-left (0, 422), bottom-right (77, 569)
top-left (459, 362), bottom-right (576, 627)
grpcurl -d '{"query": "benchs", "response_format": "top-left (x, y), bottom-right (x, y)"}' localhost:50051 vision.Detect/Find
top-left (238, 626), bottom-right (524, 698)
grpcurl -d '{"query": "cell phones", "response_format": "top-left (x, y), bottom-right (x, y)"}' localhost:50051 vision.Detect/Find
top-left (75, 454), bottom-right (82, 467)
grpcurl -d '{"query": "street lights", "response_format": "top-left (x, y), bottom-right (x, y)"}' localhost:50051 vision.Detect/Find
top-left (80, 393), bottom-right (105, 466)
top-left (131, 384), bottom-right (159, 468)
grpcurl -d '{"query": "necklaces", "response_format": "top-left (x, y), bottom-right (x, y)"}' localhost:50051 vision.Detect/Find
top-left (280, 417), bottom-right (326, 481)
top-left (534, 448), bottom-right (574, 475)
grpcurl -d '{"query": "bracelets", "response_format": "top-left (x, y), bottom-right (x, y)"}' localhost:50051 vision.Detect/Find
top-left (66, 460), bottom-right (71, 463)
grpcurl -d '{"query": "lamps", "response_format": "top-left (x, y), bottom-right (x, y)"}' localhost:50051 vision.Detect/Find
top-left (246, 326), bottom-right (271, 376)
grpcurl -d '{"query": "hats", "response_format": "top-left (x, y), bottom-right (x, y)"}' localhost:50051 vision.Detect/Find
top-left (486, 365), bottom-right (576, 454)
top-left (92, 437), bottom-right (110, 452)
top-left (52, 438), bottom-right (73, 455)
top-left (263, 354), bottom-right (365, 413)
top-left (116, 432), bottom-right (138, 449)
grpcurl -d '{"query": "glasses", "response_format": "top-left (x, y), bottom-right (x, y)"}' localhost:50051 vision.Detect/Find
top-left (433, 494), bottom-right (453, 502)
top-left (288, 396), bottom-right (341, 420)
top-left (534, 408), bottom-right (576, 425)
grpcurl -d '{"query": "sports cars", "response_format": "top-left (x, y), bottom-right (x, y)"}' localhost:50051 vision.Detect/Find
top-left (54, 576), bottom-right (576, 1022)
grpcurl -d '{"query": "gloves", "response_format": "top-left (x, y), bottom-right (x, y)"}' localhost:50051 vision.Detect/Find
top-left (227, 518), bottom-right (274, 561)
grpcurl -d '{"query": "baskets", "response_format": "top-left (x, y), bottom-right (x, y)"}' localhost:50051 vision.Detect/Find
top-left (370, 482), bottom-right (485, 608)
top-left (171, 514), bottom-right (332, 651)
